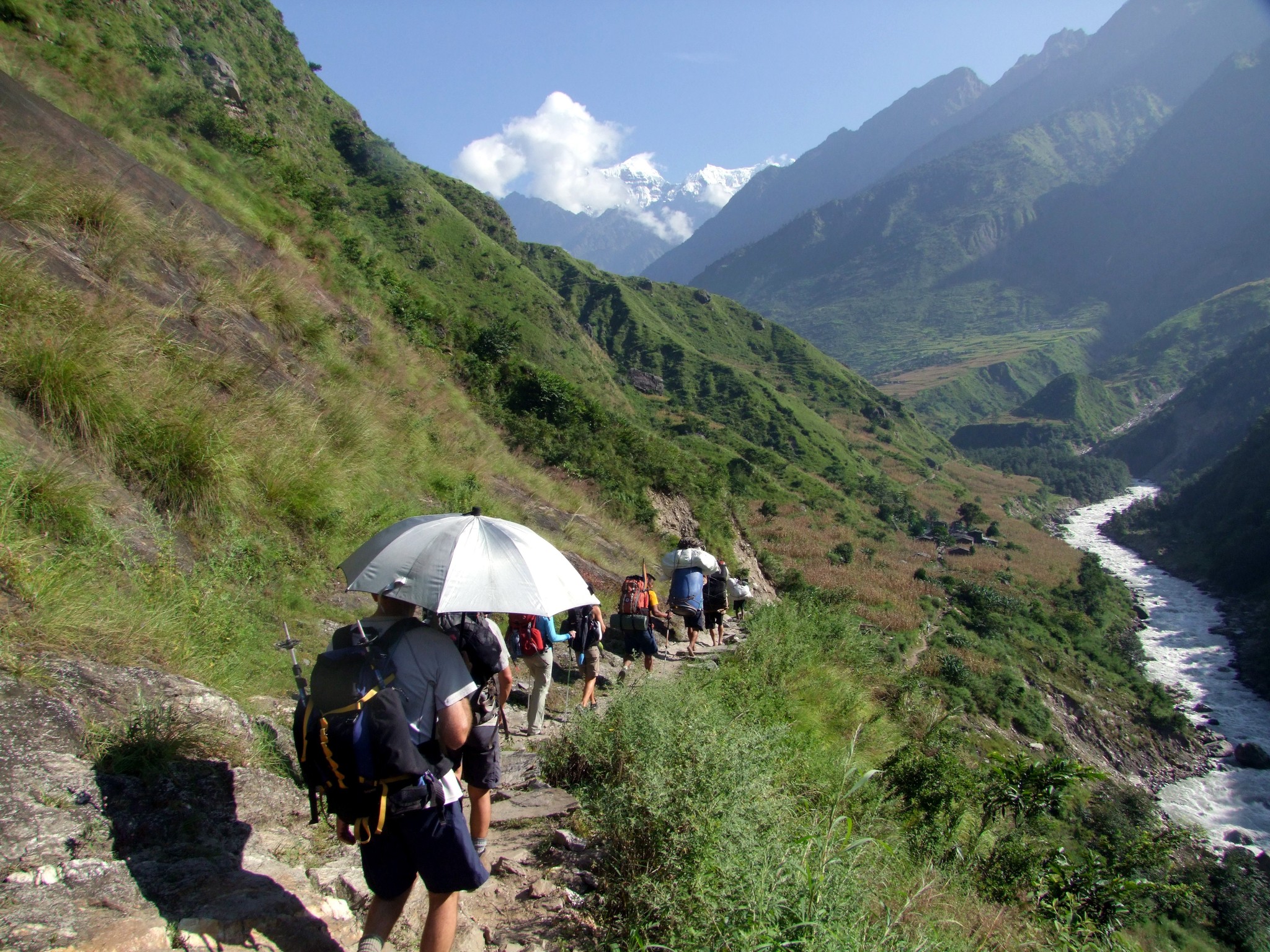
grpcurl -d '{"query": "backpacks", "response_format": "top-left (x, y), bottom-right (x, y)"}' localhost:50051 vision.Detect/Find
top-left (429, 612), bottom-right (500, 725)
top-left (678, 537), bottom-right (703, 549)
top-left (289, 616), bottom-right (444, 825)
top-left (560, 604), bottom-right (597, 653)
top-left (618, 575), bottom-right (647, 614)
top-left (505, 613), bottom-right (545, 657)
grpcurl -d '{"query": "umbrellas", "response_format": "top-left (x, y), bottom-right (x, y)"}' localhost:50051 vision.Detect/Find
top-left (337, 506), bottom-right (602, 621)
top-left (642, 557), bottom-right (648, 608)
top-left (578, 605), bottom-right (593, 665)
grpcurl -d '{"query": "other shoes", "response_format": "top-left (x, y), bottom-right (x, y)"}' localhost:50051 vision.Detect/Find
top-left (527, 729), bottom-right (546, 737)
top-left (590, 703), bottom-right (598, 709)
top-left (617, 670), bottom-right (626, 685)
top-left (576, 705), bottom-right (587, 716)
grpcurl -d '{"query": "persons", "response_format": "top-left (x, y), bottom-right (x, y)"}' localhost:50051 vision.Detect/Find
top-left (568, 583), bottom-right (606, 714)
top-left (617, 574), bottom-right (673, 686)
top-left (683, 575), bottom-right (707, 656)
top-left (705, 589), bottom-right (728, 646)
top-left (422, 610), bottom-right (513, 873)
top-left (733, 576), bottom-right (746, 620)
top-left (522, 616), bottom-right (576, 737)
top-left (326, 594), bottom-right (490, 951)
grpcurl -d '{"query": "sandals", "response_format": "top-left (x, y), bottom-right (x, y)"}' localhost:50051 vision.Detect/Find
top-left (593, 620), bottom-right (602, 642)
